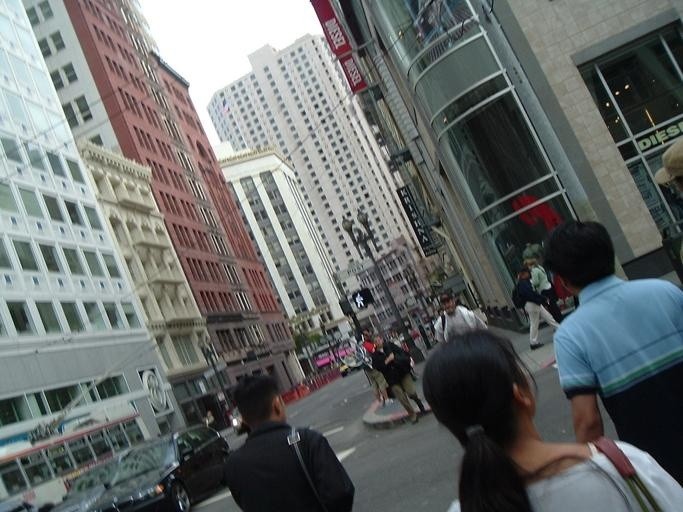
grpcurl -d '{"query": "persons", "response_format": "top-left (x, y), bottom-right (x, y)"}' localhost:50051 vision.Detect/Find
top-left (356, 290), bottom-right (488, 425)
top-left (420, 328), bottom-right (683, 512)
top-left (660, 225), bottom-right (682, 283)
top-left (516, 268), bottom-right (560, 350)
top-left (650, 135), bottom-right (682, 260)
top-left (221, 373), bottom-right (355, 511)
top-left (540, 219), bottom-right (682, 489)
top-left (521, 257), bottom-right (564, 332)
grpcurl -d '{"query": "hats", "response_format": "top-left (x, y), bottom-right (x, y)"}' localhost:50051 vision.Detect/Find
top-left (518, 267), bottom-right (529, 272)
top-left (439, 291), bottom-right (453, 302)
top-left (654, 137), bottom-right (683, 184)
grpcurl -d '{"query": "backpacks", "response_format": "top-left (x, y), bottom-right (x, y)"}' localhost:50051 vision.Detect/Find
top-left (511, 281), bottom-right (528, 309)
top-left (356, 344), bottom-right (374, 371)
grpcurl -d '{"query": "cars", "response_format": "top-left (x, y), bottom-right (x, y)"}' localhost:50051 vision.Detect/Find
top-left (340, 357), bottom-right (361, 376)
top-left (50, 424), bottom-right (232, 512)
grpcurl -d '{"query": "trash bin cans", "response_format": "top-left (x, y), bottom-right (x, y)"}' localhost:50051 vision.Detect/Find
top-left (661, 219), bottom-right (683, 284)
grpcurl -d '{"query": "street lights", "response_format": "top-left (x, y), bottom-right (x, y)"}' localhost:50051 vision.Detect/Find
top-left (342, 208), bottom-right (433, 349)
top-left (197, 337), bottom-right (235, 414)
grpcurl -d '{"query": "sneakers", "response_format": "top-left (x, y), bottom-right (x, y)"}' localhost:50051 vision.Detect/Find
top-left (410, 404), bottom-right (426, 423)
top-left (530, 342), bottom-right (545, 349)
top-left (380, 399), bottom-right (393, 408)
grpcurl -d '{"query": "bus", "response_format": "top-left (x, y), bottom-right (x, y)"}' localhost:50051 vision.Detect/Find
top-left (0, 410), bottom-right (151, 512)
